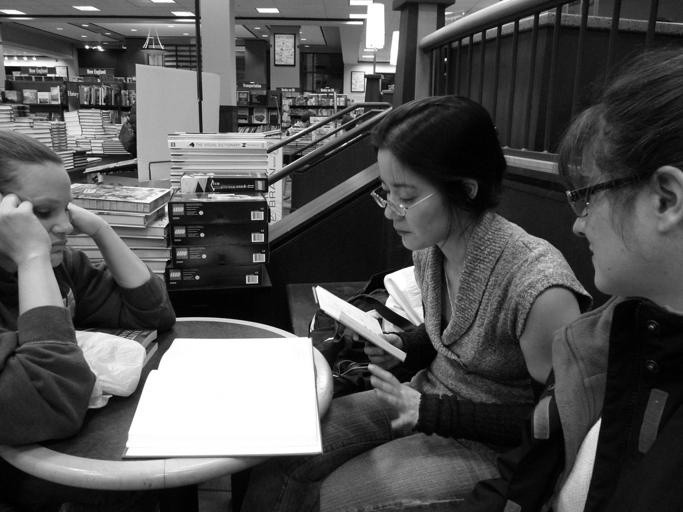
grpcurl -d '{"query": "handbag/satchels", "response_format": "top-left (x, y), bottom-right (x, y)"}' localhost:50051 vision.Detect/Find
top-left (309, 286), bottom-right (428, 392)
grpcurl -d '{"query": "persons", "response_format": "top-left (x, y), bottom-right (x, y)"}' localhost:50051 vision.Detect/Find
top-left (239, 95), bottom-right (594, 511)
top-left (0, 130), bottom-right (176, 511)
top-left (459, 45), bottom-right (683, 512)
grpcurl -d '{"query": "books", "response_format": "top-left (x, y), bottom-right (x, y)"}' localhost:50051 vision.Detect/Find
top-left (82, 328), bottom-right (159, 369)
top-left (0, 77), bottom-right (364, 288)
top-left (121, 336), bottom-right (324, 459)
top-left (311, 284), bottom-right (407, 364)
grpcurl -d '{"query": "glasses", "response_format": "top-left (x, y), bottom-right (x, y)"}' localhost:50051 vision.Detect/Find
top-left (371, 186), bottom-right (441, 218)
top-left (566, 172), bottom-right (652, 217)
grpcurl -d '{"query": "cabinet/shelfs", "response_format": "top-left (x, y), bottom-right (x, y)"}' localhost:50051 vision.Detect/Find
top-left (235, 85), bottom-right (349, 139)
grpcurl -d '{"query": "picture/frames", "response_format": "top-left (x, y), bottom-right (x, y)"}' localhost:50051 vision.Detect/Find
top-left (351, 70), bottom-right (365, 92)
top-left (274, 32), bottom-right (296, 66)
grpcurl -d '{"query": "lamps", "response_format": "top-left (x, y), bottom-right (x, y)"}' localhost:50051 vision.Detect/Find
top-left (138, 25), bottom-right (170, 56)
top-left (366, 2), bottom-right (386, 73)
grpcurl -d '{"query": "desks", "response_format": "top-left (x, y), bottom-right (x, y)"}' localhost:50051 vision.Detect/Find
top-left (0, 317), bottom-right (335, 511)
